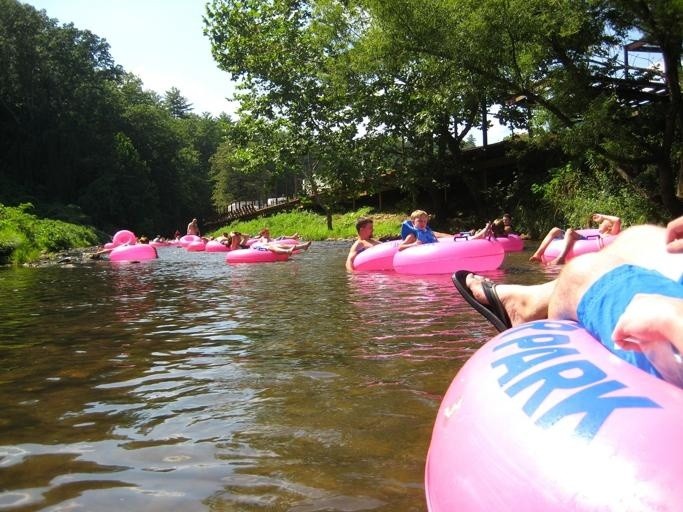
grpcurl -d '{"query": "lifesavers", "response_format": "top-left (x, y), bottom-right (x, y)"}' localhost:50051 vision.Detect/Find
top-left (541, 228), bottom-right (616, 264)
top-left (424, 320), bottom-right (681, 511)
top-left (354, 239), bottom-right (405, 271)
top-left (393, 237), bottom-right (504, 275)
top-left (105, 230), bottom-right (136, 249)
top-left (109, 245), bottom-right (155, 261)
top-left (150, 235), bottom-right (301, 264)
top-left (494, 235), bottom-right (523, 251)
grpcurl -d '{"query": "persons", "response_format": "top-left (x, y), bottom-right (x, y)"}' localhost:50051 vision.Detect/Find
top-left (529, 214), bottom-right (621, 266)
top-left (451, 215), bottom-right (683, 389)
top-left (345, 218), bottom-right (475, 273)
top-left (97, 218), bottom-right (311, 256)
top-left (475, 213), bottom-right (512, 237)
top-left (398, 209), bottom-right (493, 251)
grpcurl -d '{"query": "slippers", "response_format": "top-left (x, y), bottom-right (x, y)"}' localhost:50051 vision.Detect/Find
top-left (452, 270), bottom-right (511, 333)
